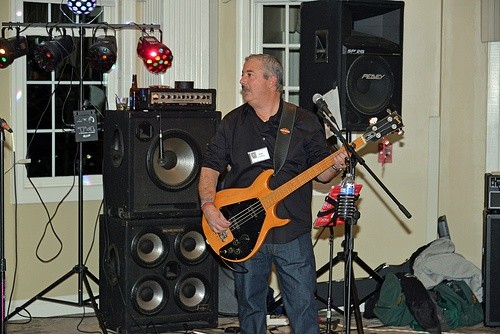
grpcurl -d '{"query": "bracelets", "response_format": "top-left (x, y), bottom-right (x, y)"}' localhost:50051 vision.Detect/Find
top-left (332, 165), bottom-right (342, 172)
top-left (200, 199), bottom-right (216, 211)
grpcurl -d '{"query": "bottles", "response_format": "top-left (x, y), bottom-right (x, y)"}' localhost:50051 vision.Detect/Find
top-left (338, 173), bottom-right (355, 221)
top-left (130, 73), bottom-right (139, 111)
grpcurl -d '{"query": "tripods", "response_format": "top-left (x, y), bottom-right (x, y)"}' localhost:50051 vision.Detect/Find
top-left (4, 28), bottom-right (107, 334)
top-left (266, 133), bottom-right (384, 334)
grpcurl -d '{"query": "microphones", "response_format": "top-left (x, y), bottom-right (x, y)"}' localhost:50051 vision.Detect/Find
top-left (312, 92), bottom-right (335, 122)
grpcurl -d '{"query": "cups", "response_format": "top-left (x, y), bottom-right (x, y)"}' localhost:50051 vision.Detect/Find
top-left (116, 97), bottom-right (129, 111)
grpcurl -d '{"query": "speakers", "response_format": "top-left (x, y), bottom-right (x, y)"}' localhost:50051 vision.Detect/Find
top-left (299, 0), bottom-right (404, 132)
top-left (99, 214), bottom-right (219, 334)
top-left (102, 110), bottom-right (222, 219)
top-left (484, 212), bottom-right (500, 327)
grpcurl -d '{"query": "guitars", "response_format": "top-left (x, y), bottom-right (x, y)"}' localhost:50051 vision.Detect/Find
top-left (202, 108), bottom-right (406, 262)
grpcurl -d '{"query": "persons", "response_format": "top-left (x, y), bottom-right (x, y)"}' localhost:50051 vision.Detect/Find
top-left (197, 53), bottom-right (352, 334)
